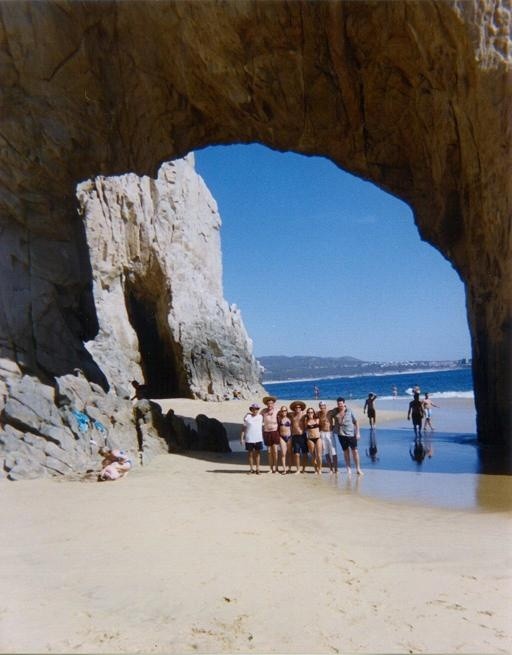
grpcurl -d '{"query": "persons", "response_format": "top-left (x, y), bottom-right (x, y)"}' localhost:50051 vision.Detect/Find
top-left (392, 385), bottom-right (398, 400)
top-left (407, 393), bottom-right (424, 430)
top-left (313, 384), bottom-right (320, 400)
top-left (365, 430), bottom-right (381, 463)
top-left (363, 392), bottom-right (378, 429)
top-left (237, 395), bottom-right (365, 477)
top-left (420, 392), bottom-right (441, 430)
top-left (413, 383), bottom-right (420, 395)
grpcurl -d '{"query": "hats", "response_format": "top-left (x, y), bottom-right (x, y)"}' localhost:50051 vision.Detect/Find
top-left (249, 403), bottom-right (261, 411)
top-left (319, 401), bottom-right (326, 406)
top-left (290, 401), bottom-right (306, 411)
top-left (262, 397), bottom-right (276, 405)
